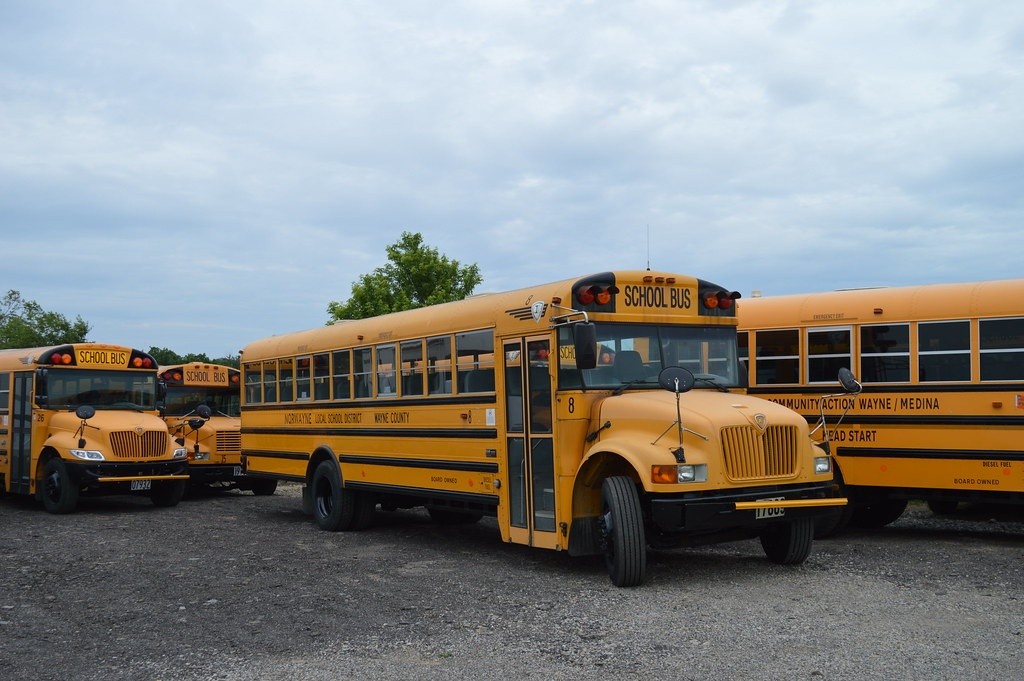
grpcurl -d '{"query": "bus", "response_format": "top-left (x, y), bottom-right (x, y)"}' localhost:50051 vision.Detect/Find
top-left (238, 268), bottom-right (864, 590)
top-left (0, 342), bottom-right (212, 514)
top-left (633, 277), bottom-right (1024, 536)
top-left (53, 361), bottom-right (280, 495)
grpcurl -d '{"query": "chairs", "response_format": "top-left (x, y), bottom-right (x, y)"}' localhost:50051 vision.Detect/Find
top-left (167, 402), bottom-right (216, 415)
top-left (252, 363), bottom-right (581, 428)
top-left (613, 349), bottom-right (647, 382)
